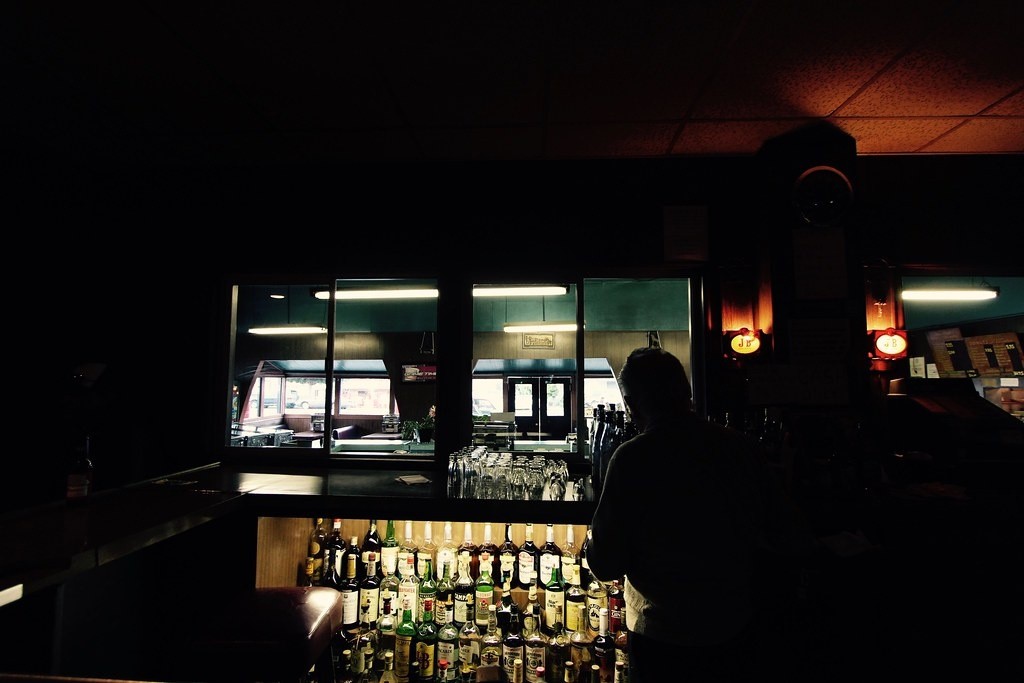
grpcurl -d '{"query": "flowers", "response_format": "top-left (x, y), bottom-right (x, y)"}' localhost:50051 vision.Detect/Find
top-left (401, 404), bottom-right (436, 440)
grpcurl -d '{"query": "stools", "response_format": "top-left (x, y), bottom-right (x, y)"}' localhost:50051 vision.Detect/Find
top-left (191, 586), bottom-right (344, 683)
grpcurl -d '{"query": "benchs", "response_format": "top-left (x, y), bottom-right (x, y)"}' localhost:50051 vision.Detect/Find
top-left (332, 425), bottom-right (358, 440)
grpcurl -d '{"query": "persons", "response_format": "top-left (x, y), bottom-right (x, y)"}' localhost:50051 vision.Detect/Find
top-left (588, 350), bottom-right (812, 683)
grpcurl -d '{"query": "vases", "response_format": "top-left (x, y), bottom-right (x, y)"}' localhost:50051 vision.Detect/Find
top-left (414, 428), bottom-right (433, 445)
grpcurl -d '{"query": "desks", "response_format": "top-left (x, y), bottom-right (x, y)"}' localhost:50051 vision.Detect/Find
top-left (0, 460), bottom-right (446, 683)
top-left (361, 432), bottom-right (402, 441)
top-left (292, 430), bottom-right (324, 448)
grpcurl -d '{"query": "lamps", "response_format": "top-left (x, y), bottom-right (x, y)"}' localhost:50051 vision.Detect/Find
top-left (314, 281), bottom-right (571, 301)
top-left (503, 285), bottom-right (586, 333)
top-left (243, 287), bottom-right (328, 336)
top-left (900, 278), bottom-right (1001, 302)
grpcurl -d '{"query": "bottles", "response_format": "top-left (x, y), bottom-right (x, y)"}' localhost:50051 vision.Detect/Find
top-left (448, 445), bottom-right (586, 501)
top-left (304, 518), bottom-right (629, 683)
top-left (66, 435), bottom-right (95, 507)
top-left (710, 408), bottom-right (781, 448)
top-left (589, 404), bottom-right (633, 482)
